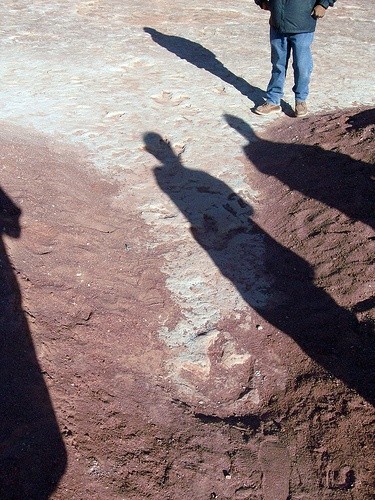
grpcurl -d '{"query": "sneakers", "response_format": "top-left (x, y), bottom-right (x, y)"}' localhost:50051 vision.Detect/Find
top-left (254, 101), bottom-right (282, 115)
top-left (295, 99), bottom-right (307, 117)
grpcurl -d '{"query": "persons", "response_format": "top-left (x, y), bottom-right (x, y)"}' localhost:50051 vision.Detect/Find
top-left (253, 0), bottom-right (337, 118)
